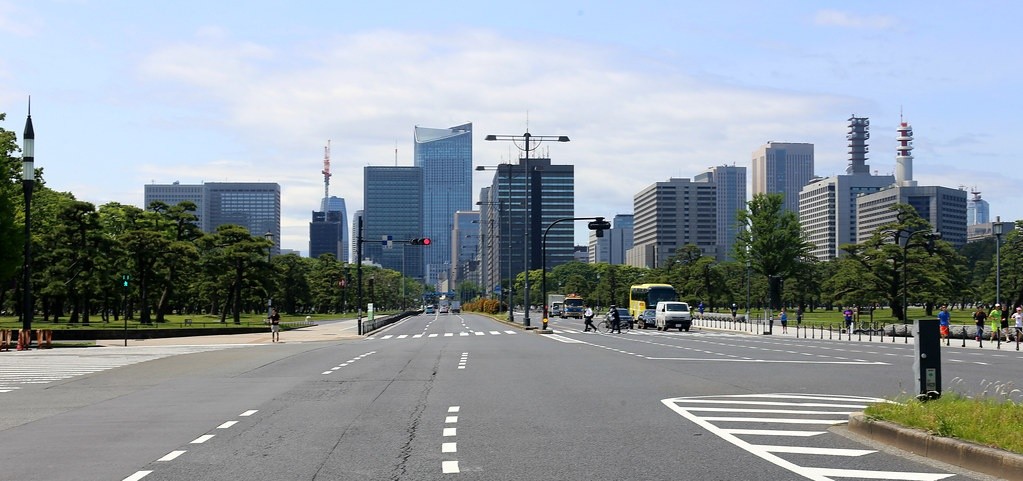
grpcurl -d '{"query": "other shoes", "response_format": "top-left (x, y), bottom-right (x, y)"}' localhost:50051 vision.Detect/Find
top-left (594, 328), bottom-right (597, 331)
top-left (1014, 336), bottom-right (1017, 342)
top-left (277, 338), bottom-right (279, 341)
top-left (990, 337), bottom-right (993, 343)
top-left (1006, 340), bottom-right (1011, 343)
top-left (617, 332), bottom-right (621, 334)
top-left (583, 330), bottom-right (587, 332)
top-left (975, 337), bottom-right (979, 341)
top-left (609, 331), bottom-right (613, 333)
top-left (272, 340), bottom-right (274, 342)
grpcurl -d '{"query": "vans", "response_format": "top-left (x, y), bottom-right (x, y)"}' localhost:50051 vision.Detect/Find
top-left (655, 302), bottom-right (692, 332)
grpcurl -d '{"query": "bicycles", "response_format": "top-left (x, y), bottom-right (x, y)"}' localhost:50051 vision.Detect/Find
top-left (596, 314), bottom-right (630, 335)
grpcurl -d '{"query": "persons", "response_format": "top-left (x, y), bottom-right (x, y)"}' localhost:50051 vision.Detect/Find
top-left (938, 305), bottom-right (950, 342)
top-left (1011, 307), bottom-right (1023, 344)
top-left (609, 305), bottom-right (620, 334)
top-left (583, 304), bottom-right (597, 333)
top-left (688, 306), bottom-right (695, 319)
top-left (973, 307), bottom-right (986, 341)
top-left (1001, 304), bottom-right (1010, 343)
top-left (843, 305), bottom-right (853, 334)
top-left (795, 306), bottom-right (802, 328)
top-left (270, 309), bottom-right (279, 342)
top-left (989, 303), bottom-right (1002, 345)
top-left (731, 304), bottom-right (738, 319)
top-left (698, 302), bottom-right (704, 315)
top-left (778, 308), bottom-right (787, 334)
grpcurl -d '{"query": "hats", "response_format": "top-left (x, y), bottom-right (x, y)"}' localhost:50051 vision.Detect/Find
top-left (977, 304), bottom-right (983, 309)
top-left (1016, 307), bottom-right (1022, 311)
top-left (995, 303), bottom-right (1000, 306)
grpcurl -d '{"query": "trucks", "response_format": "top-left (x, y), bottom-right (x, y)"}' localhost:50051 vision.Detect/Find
top-left (450, 301), bottom-right (461, 313)
top-left (547, 294), bottom-right (566, 318)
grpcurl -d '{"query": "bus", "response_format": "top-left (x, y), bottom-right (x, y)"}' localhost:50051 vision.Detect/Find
top-left (629, 283), bottom-right (680, 323)
top-left (562, 295), bottom-right (584, 319)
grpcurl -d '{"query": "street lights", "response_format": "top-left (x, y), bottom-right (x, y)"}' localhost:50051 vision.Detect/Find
top-left (264, 229), bottom-right (274, 319)
top-left (476, 200), bottom-right (529, 314)
top-left (453, 218), bottom-right (507, 301)
top-left (486, 133), bottom-right (571, 326)
top-left (122, 273), bottom-right (129, 345)
top-left (992, 216), bottom-right (1003, 303)
top-left (475, 165), bottom-right (546, 323)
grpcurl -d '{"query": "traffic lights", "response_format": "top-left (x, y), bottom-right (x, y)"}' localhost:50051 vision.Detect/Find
top-left (923, 232), bottom-right (941, 239)
top-left (122, 280), bottom-right (128, 288)
top-left (411, 238), bottom-right (430, 245)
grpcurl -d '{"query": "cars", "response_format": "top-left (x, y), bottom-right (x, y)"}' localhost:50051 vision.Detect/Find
top-left (604, 309), bottom-right (634, 330)
top-left (439, 306), bottom-right (448, 313)
top-left (637, 309), bottom-right (656, 330)
top-left (426, 305), bottom-right (435, 314)
